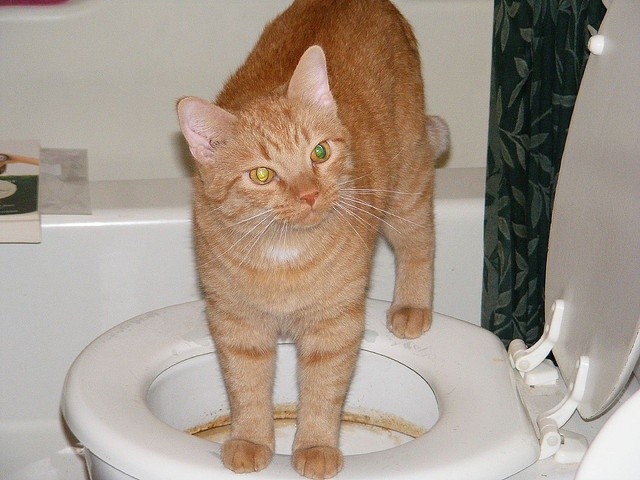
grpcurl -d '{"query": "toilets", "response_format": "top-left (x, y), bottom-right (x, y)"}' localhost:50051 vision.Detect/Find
top-left (61, 1), bottom-right (639, 480)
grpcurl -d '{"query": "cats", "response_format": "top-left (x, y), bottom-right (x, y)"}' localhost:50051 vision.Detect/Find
top-left (175, 1), bottom-right (453, 480)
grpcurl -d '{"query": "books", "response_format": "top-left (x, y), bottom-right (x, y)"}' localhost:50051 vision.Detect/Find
top-left (0, 175), bottom-right (40, 243)
